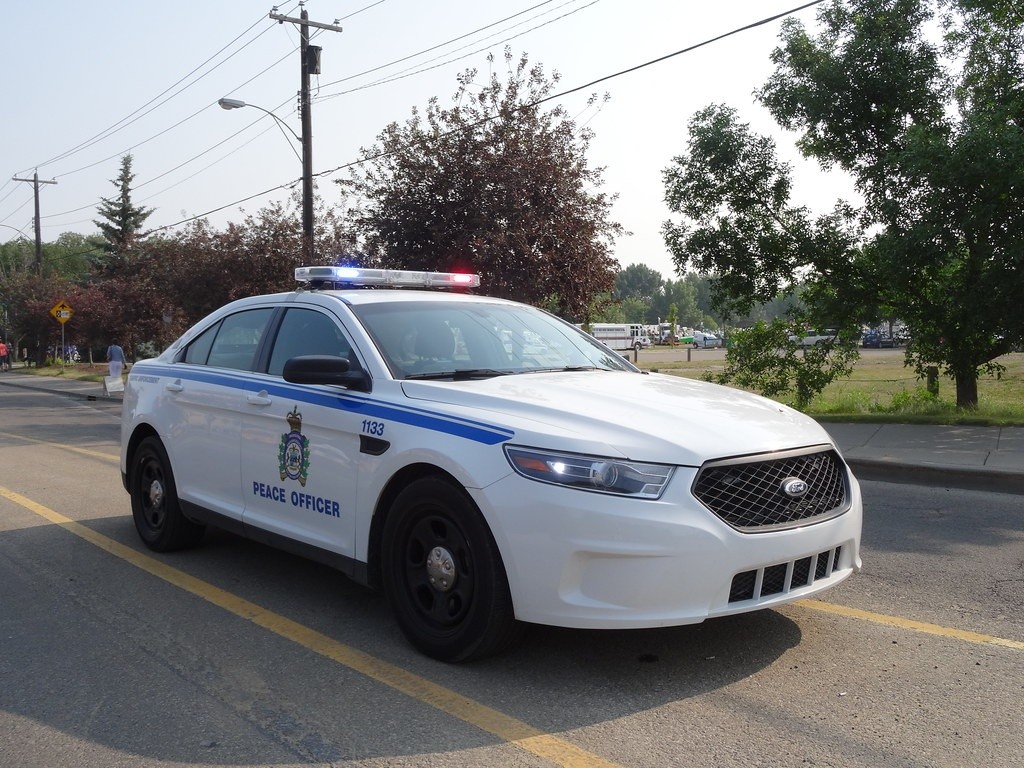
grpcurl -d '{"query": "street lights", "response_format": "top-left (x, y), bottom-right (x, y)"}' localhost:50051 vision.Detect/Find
top-left (218, 97), bottom-right (315, 267)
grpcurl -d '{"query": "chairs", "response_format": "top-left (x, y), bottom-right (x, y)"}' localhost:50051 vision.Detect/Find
top-left (412, 321), bottom-right (469, 374)
top-left (295, 321), bottom-right (342, 358)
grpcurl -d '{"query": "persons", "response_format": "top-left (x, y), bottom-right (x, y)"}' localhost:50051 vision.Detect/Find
top-left (106, 339), bottom-right (128, 377)
top-left (0, 340), bottom-right (12, 372)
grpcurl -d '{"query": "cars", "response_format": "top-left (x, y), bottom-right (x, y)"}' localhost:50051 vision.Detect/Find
top-left (861, 333), bottom-right (897, 349)
top-left (693, 333), bottom-right (723, 350)
top-left (788, 328), bottom-right (841, 347)
top-left (119, 268), bottom-right (863, 667)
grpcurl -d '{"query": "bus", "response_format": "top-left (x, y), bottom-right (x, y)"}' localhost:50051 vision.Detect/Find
top-left (647, 320), bottom-right (700, 345)
top-left (571, 322), bottom-right (651, 353)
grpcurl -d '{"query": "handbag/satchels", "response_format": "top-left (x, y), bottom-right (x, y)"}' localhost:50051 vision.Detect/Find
top-left (106, 346), bottom-right (112, 362)
